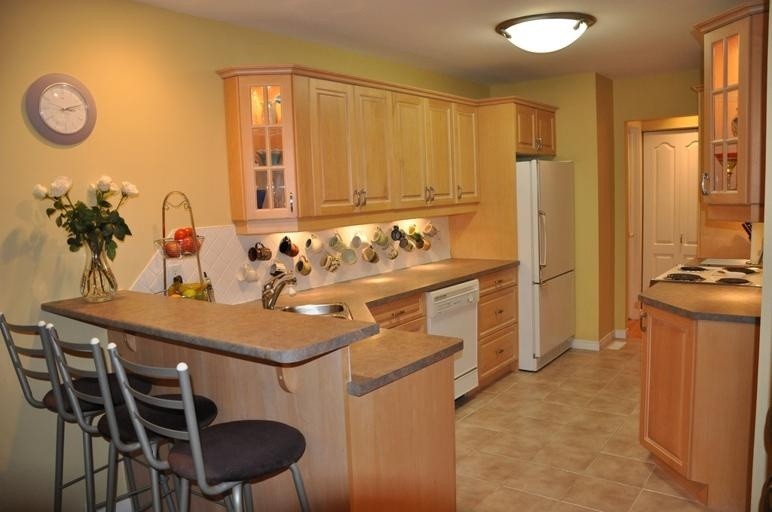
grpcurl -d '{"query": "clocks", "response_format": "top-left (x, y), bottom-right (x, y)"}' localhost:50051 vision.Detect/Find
top-left (26, 72), bottom-right (97, 145)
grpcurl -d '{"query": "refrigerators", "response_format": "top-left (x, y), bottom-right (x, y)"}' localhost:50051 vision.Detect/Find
top-left (517, 159), bottom-right (574, 372)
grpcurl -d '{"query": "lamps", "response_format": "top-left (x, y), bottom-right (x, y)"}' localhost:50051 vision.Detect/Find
top-left (495, 12), bottom-right (597, 55)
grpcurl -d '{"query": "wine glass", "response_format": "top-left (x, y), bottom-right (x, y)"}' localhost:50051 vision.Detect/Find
top-left (715, 153), bottom-right (738, 191)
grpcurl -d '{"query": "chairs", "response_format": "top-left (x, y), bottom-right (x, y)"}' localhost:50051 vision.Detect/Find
top-left (46, 322), bottom-right (231, 512)
top-left (1, 313), bottom-right (176, 511)
top-left (107, 342), bottom-right (310, 512)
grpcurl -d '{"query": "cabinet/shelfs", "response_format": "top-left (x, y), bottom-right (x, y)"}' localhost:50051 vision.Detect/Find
top-left (453, 94), bottom-right (480, 214)
top-left (695, 1), bottom-right (768, 226)
top-left (478, 95), bottom-right (559, 157)
top-left (393, 85), bottom-right (453, 220)
top-left (310, 69), bottom-right (393, 231)
top-left (477, 266), bottom-right (518, 391)
top-left (369, 294), bottom-right (428, 336)
top-left (636, 304), bottom-right (759, 512)
top-left (216, 64), bottom-right (315, 236)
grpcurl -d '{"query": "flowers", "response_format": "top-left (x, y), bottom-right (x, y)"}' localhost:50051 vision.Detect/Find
top-left (32, 176), bottom-right (139, 297)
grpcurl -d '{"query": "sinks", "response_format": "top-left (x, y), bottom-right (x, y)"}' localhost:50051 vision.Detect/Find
top-left (284, 304), bottom-right (349, 317)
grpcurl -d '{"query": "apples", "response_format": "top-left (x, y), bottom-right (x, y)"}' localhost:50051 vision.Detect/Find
top-left (183, 289), bottom-right (196, 298)
top-left (166, 227), bottom-right (196, 257)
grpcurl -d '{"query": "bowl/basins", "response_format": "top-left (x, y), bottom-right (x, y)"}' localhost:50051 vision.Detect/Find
top-left (258, 148), bottom-right (282, 165)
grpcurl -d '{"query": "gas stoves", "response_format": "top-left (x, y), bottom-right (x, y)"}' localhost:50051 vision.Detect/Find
top-left (661, 265), bottom-right (758, 285)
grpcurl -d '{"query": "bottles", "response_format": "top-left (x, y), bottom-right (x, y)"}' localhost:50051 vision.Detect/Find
top-left (260, 102), bottom-right (277, 125)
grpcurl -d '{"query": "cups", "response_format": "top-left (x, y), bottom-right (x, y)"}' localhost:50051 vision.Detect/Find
top-left (237, 223), bottom-right (437, 282)
top-left (256, 187), bottom-right (267, 209)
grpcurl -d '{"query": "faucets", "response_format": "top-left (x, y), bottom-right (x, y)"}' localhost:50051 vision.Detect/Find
top-left (260, 270), bottom-right (297, 308)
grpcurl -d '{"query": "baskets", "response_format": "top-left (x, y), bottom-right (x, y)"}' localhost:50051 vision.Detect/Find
top-left (154, 235), bottom-right (204, 259)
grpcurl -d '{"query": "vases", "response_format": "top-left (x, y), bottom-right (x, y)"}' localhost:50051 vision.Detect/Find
top-left (79, 239), bottom-right (118, 303)
